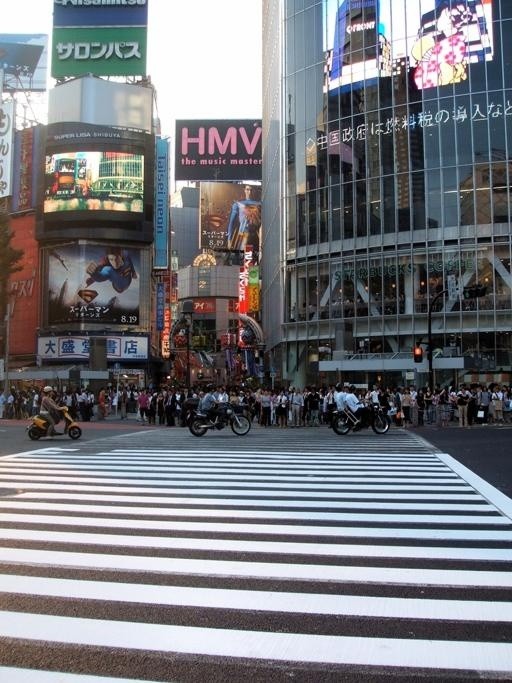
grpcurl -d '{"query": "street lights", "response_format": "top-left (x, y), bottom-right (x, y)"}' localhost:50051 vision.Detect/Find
top-left (180, 299), bottom-right (196, 391)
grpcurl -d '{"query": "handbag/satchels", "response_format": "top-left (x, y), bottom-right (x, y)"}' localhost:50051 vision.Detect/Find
top-left (396, 412), bottom-right (405, 419)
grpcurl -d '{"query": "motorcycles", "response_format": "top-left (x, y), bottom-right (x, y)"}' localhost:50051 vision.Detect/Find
top-left (330, 396), bottom-right (391, 435)
top-left (25, 398), bottom-right (83, 441)
top-left (183, 397), bottom-right (250, 436)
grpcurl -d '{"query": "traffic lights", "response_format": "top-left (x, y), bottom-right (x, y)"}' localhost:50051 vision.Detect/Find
top-left (462, 286), bottom-right (487, 299)
top-left (412, 347), bottom-right (423, 364)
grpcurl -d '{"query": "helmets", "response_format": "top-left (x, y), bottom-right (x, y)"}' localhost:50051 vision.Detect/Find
top-left (336, 383), bottom-right (343, 392)
top-left (206, 383), bottom-right (214, 391)
top-left (44, 386), bottom-right (52, 392)
top-left (349, 385), bottom-right (356, 393)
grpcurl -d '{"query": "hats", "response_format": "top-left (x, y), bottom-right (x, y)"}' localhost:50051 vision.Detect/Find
top-left (239, 391), bottom-right (245, 394)
top-left (295, 388), bottom-right (301, 392)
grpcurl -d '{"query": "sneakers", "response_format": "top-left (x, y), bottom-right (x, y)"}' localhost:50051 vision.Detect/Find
top-left (47, 430), bottom-right (56, 436)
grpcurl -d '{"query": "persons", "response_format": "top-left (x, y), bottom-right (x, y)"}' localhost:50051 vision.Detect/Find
top-left (85, 247), bottom-right (138, 294)
top-left (227, 185), bottom-right (261, 250)
